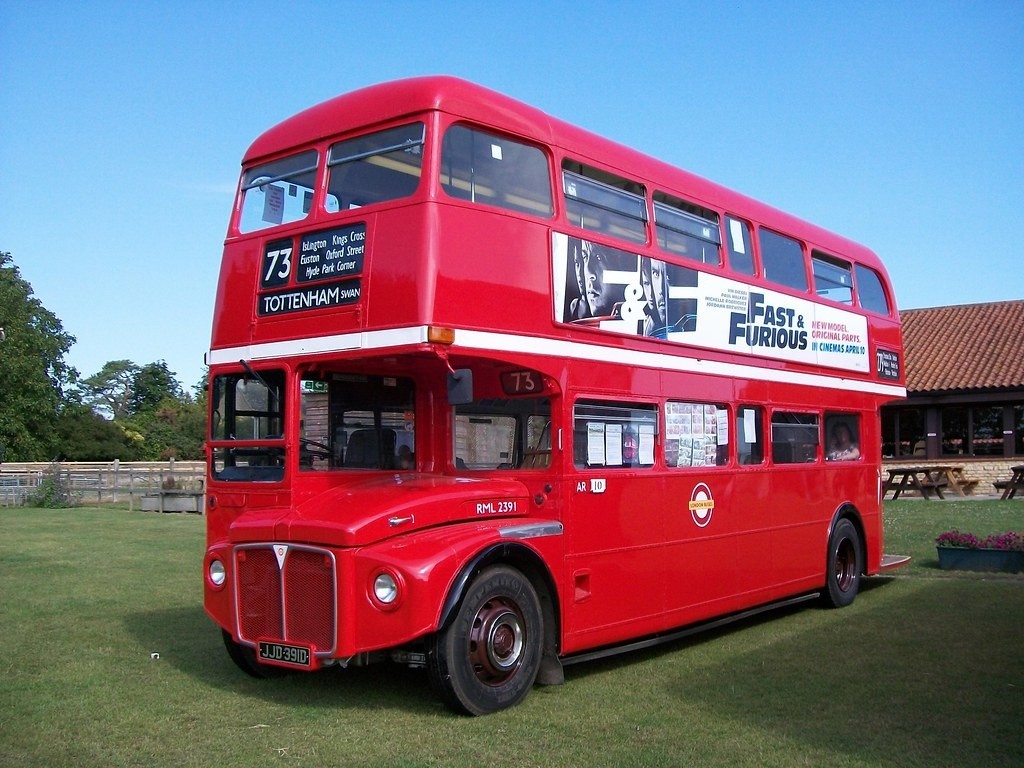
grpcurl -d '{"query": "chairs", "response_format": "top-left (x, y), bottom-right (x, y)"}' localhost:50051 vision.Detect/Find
top-left (344, 427), bottom-right (399, 469)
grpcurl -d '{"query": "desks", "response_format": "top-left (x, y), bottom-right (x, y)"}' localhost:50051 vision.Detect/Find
top-left (993, 464), bottom-right (1024, 501)
top-left (914, 464), bottom-right (982, 498)
top-left (881, 467), bottom-right (951, 502)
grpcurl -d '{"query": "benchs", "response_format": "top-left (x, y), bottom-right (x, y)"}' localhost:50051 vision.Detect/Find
top-left (884, 438), bottom-right (1005, 455)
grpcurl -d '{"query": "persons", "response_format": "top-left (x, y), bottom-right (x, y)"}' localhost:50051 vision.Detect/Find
top-left (569, 243), bottom-right (591, 321)
top-left (582, 241), bottom-right (627, 317)
top-left (826, 422), bottom-right (861, 461)
top-left (640, 255), bottom-right (656, 316)
top-left (644, 257), bottom-right (690, 341)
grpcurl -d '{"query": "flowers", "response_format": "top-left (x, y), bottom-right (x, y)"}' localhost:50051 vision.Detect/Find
top-left (935, 529), bottom-right (1024, 550)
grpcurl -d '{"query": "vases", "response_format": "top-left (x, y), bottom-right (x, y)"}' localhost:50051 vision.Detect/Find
top-left (936, 546), bottom-right (1024, 573)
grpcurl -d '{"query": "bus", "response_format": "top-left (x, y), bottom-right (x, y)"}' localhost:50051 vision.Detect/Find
top-left (196, 75), bottom-right (910, 721)
